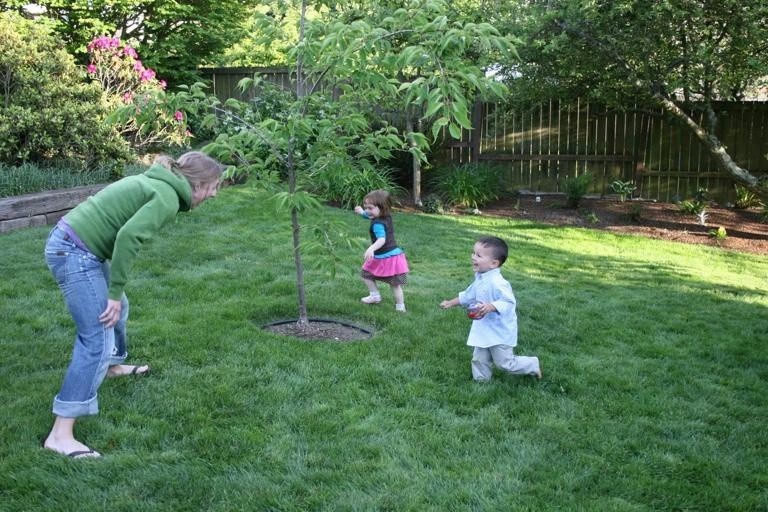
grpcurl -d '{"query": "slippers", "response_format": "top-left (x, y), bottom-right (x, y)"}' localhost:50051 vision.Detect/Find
top-left (120, 365), bottom-right (152, 376)
top-left (44, 442), bottom-right (102, 458)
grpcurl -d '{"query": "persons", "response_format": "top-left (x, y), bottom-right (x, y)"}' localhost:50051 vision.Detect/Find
top-left (438, 237), bottom-right (542, 382)
top-left (44, 152), bottom-right (223, 459)
top-left (354, 189), bottom-right (409, 314)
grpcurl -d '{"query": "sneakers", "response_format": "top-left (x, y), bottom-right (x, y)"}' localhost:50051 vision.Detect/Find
top-left (396, 303), bottom-right (407, 313)
top-left (361, 295), bottom-right (382, 303)
